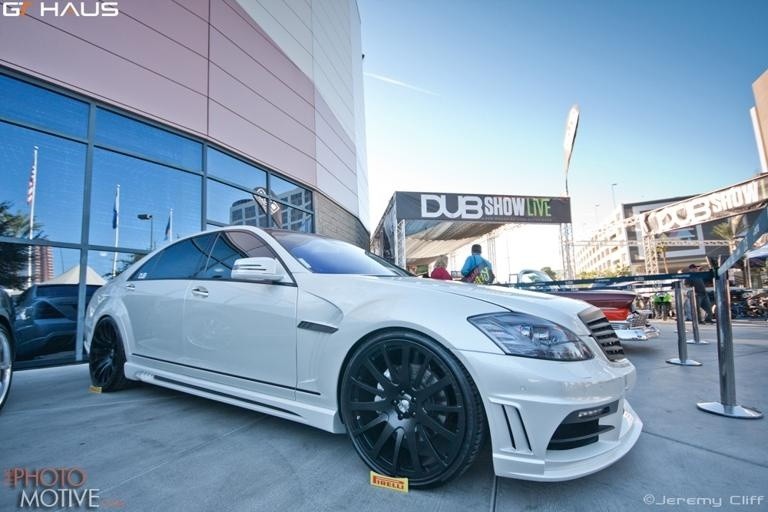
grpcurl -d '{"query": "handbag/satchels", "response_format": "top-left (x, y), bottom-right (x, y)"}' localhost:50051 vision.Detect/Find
top-left (459, 253), bottom-right (495, 286)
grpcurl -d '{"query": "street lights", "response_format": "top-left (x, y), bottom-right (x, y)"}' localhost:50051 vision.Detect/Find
top-left (594, 204), bottom-right (599, 226)
top-left (136, 213), bottom-right (155, 253)
top-left (610, 181), bottom-right (618, 212)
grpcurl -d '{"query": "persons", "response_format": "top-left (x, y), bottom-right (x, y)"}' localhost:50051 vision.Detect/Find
top-left (431, 255), bottom-right (453, 280)
top-left (460, 244), bottom-right (495, 285)
top-left (684, 264), bottom-right (717, 324)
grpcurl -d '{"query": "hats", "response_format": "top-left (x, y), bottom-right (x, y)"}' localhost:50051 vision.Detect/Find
top-left (688, 264), bottom-right (700, 269)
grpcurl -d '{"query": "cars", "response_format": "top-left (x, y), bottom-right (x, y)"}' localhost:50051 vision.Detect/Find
top-left (641, 284), bottom-right (767, 323)
top-left (515, 268), bottom-right (662, 341)
top-left (14, 282), bottom-right (104, 356)
top-left (0, 284), bottom-right (15, 410)
top-left (79, 224), bottom-right (646, 483)
